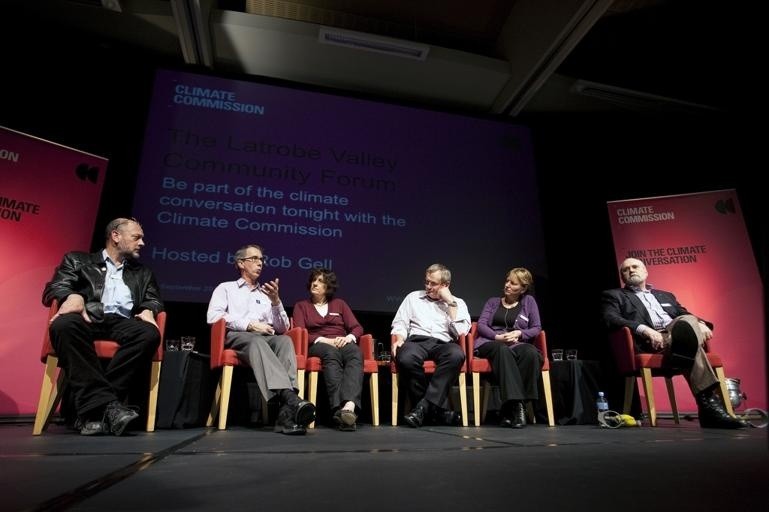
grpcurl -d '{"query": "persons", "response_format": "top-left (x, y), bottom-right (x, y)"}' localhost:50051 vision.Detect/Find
top-left (390, 264), bottom-right (472, 428)
top-left (473, 267), bottom-right (543, 429)
top-left (293, 268), bottom-right (365, 432)
top-left (207, 244), bottom-right (315, 436)
top-left (601, 257), bottom-right (747, 429)
top-left (41, 218), bottom-right (164, 435)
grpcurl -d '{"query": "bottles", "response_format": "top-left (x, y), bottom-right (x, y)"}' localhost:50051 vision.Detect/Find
top-left (596, 392), bottom-right (610, 428)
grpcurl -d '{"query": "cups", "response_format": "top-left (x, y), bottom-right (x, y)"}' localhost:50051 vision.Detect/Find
top-left (166, 339), bottom-right (180, 352)
top-left (181, 336), bottom-right (196, 350)
top-left (551, 348), bottom-right (563, 361)
top-left (565, 349), bottom-right (578, 360)
top-left (380, 350), bottom-right (391, 361)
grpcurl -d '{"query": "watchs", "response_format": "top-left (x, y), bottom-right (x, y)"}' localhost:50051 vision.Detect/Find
top-left (448, 302), bottom-right (457, 307)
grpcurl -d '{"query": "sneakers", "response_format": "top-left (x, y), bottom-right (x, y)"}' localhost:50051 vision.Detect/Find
top-left (74, 400), bottom-right (139, 436)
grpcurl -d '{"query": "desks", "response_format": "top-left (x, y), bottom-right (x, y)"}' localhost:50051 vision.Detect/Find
top-left (154, 347), bottom-right (217, 430)
top-left (532, 358), bottom-right (595, 426)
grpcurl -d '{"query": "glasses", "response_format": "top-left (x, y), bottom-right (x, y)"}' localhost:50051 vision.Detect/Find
top-left (237, 256), bottom-right (266, 263)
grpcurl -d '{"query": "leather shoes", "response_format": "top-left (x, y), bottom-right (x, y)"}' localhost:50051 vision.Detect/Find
top-left (441, 410), bottom-right (461, 426)
top-left (273, 395), bottom-right (316, 434)
top-left (500, 401), bottom-right (526, 429)
top-left (695, 395), bottom-right (747, 430)
top-left (404, 406), bottom-right (423, 428)
top-left (668, 320), bottom-right (698, 375)
top-left (333, 408), bottom-right (358, 432)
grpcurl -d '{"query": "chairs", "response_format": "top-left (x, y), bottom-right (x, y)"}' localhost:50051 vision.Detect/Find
top-left (31, 298), bottom-right (169, 436)
top-left (608, 324), bottom-right (736, 428)
top-left (289, 315), bottom-right (382, 429)
top-left (390, 332), bottom-right (470, 429)
top-left (466, 320), bottom-right (558, 430)
top-left (205, 318), bottom-right (311, 430)
